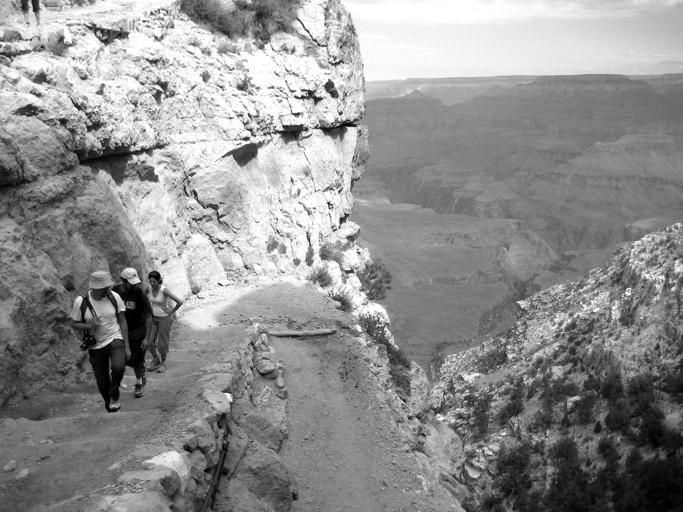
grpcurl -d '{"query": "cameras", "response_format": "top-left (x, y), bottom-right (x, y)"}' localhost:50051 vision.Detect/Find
top-left (80, 334), bottom-right (96, 351)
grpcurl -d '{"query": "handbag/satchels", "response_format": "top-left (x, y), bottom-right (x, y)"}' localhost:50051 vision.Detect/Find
top-left (69, 317), bottom-right (88, 341)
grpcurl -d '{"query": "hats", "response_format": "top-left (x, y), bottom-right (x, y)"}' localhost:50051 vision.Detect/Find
top-left (89, 271), bottom-right (113, 290)
top-left (120, 267), bottom-right (142, 285)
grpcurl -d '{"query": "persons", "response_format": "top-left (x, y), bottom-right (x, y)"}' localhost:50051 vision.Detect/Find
top-left (69, 267), bottom-right (183, 413)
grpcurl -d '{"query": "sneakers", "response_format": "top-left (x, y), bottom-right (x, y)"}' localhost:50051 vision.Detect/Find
top-left (105, 396), bottom-right (121, 412)
top-left (146, 358), bottom-right (168, 373)
top-left (134, 377), bottom-right (147, 398)
